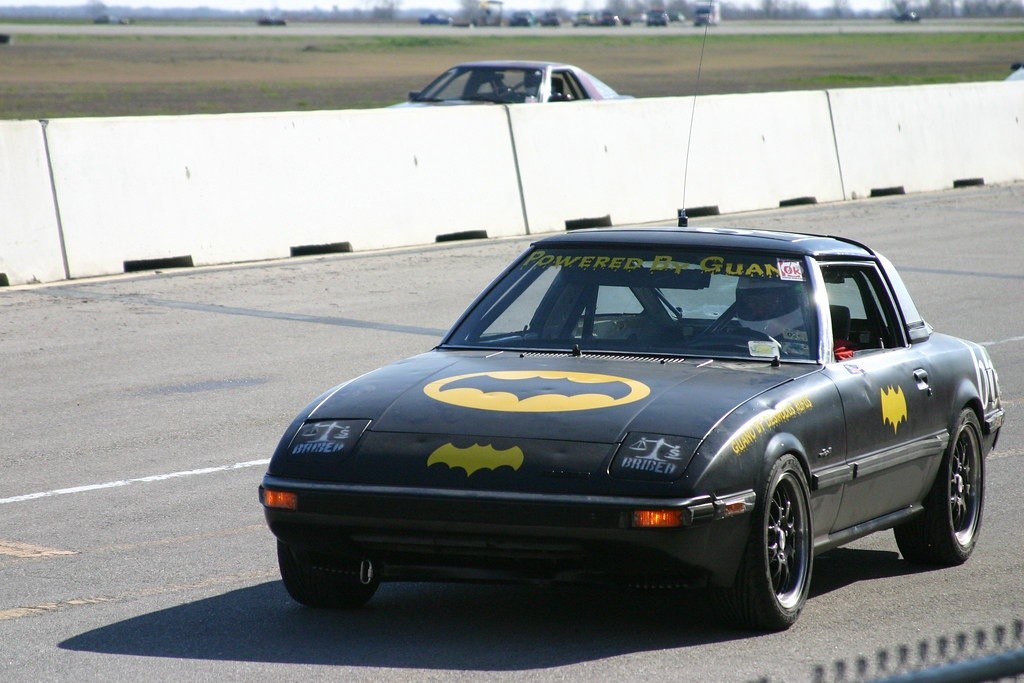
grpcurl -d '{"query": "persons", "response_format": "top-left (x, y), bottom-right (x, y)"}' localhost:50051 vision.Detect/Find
top-left (703, 274), bottom-right (810, 357)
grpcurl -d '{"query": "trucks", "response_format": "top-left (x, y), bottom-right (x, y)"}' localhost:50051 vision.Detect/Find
top-left (694, 2), bottom-right (721, 27)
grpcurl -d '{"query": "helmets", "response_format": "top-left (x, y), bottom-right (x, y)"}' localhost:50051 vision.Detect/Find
top-left (524, 69), bottom-right (553, 95)
top-left (735, 275), bottom-right (804, 339)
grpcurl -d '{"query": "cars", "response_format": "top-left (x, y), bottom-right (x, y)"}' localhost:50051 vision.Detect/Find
top-left (892, 10), bottom-right (921, 22)
top-left (417, 10), bottom-right (631, 30)
top-left (260, 212), bottom-right (1005, 637)
top-left (254, 16), bottom-right (288, 28)
top-left (646, 9), bottom-right (670, 25)
top-left (382, 60), bottom-right (637, 109)
